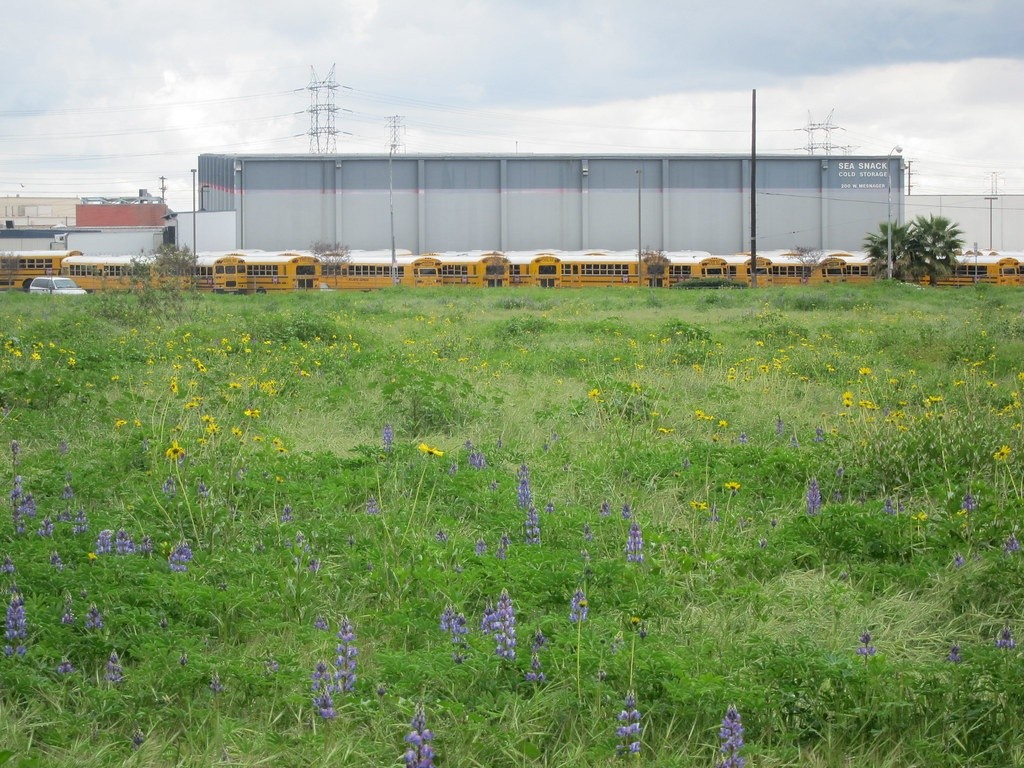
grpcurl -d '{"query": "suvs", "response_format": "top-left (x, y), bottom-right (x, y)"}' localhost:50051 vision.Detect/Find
top-left (30, 276), bottom-right (88, 295)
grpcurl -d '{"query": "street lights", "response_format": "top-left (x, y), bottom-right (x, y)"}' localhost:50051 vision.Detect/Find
top-left (885, 144), bottom-right (904, 279)
